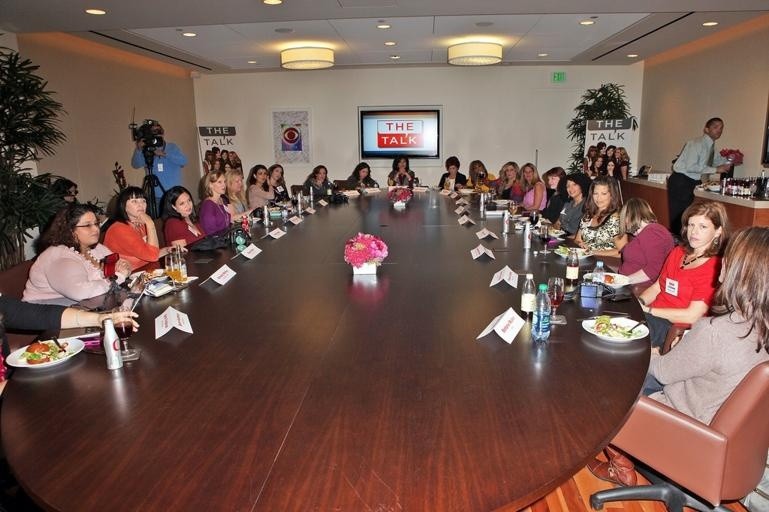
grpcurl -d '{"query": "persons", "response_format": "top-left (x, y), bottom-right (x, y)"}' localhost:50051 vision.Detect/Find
top-left (439, 156), bottom-right (768, 488)
top-left (202, 145), bottom-right (242, 173)
top-left (668, 117), bottom-right (735, 233)
top-left (585, 141), bottom-right (632, 181)
top-left (130, 119), bottom-right (187, 217)
top-left (1, 158), bottom-right (291, 393)
top-left (302, 158), bottom-right (415, 200)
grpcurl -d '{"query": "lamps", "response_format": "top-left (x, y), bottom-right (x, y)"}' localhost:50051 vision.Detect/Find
top-left (448, 41), bottom-right (504, 69)
top-left (277, 46), bottom-right (336, 73)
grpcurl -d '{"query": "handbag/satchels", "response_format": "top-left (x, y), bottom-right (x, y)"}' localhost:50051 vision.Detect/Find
top-left (249, 208), bottom-right (270, 218)
top-left (191, 235), bottom-right (228, 250)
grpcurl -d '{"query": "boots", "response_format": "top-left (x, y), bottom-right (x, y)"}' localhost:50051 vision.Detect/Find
top-left (586, 446), bottom-right (637, 487)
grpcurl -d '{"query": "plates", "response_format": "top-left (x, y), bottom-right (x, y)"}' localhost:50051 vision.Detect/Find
top-left (576, 266), bottom-right (634, 291)
top-left (3, 352), bottom-right (94, 386)
top-left (127, 265), bottom-right (200, 296)
top-left (553, 246), bottom-right (594, 260)
top-left (5, 337), bottom-right (88, 371)
top-left (579, 313), bottom-right (652, 345)
top-left (581, 331), bottom-right (647, 356)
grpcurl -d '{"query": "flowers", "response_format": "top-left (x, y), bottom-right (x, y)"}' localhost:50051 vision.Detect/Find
top-left (388, 187), bottom-right (414, 201)
top-left (341, 230), bottom-right (389, 268)
top-left (720, 147), bottom-right (744, 166)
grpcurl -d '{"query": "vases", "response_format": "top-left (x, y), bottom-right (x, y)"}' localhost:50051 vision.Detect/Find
top-left (350, 261), bottom-right (378, 275)
top-left (719, 165), bottom-right (735, 181)
top-left (393, 200), bottom-right (405, 208)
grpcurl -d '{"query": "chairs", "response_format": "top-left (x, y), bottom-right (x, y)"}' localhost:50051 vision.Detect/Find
top-left (585, 356), bottom-right (768, 512)
top-left (2, 179), bottom-right (355, 354)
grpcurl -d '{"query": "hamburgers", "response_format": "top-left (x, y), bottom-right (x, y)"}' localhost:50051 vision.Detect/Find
top-left (19, 343), bottom-right (50, 364)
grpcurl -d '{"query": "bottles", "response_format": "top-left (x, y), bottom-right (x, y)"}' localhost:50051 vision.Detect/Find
top-left (531, 284), bottom-right (552, 342)
top-left (226, 179), bottom-right (575, 250)
top-left (100, 316), bottom-right (125, 373)
top-left (695, 153), bottom-right (769, 199)
top-left (174, 244), bottom-right (191, 286)
top-left (516, 319), bottom-right (532, 346)
top-left (519, 272), bottom-right (539, 322)
top-left (589, 258), bottom-right (605, 297)
top-left (563, 247), bottom-right (582, 302)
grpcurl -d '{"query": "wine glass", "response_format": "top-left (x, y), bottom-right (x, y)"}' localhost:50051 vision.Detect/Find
top-left (161, 254), bottom-right (180, 293)
top-left (109, 303), bottom-right (143, 364)
top-left (537, 226), bottom-right (552, 253)
top-left (544, 276), bottom-right (569, 329)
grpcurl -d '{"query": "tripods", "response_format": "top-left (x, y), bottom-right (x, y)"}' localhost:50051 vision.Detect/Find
top-left (141, 175), bottom-right (166, 220)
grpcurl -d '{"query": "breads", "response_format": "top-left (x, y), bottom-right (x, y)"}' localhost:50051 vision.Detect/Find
top-left (605, 275), bottom-right (613, 283)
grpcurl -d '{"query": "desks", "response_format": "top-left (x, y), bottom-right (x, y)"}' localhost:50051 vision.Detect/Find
top-left (691, 185), bottom-right (769, 234)
top-left (623, 174), bottom-right (671, 232)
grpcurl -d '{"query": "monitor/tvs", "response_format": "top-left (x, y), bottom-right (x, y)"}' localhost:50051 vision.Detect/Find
top-left (361, 110), bottom-right (440, 158)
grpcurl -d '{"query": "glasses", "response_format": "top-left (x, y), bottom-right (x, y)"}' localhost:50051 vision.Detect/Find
top-left (76, 222), bottom-right (100, 227)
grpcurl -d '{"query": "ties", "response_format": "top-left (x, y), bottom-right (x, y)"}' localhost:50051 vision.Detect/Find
top-left (708, 143), bottom-right (715, 167)
